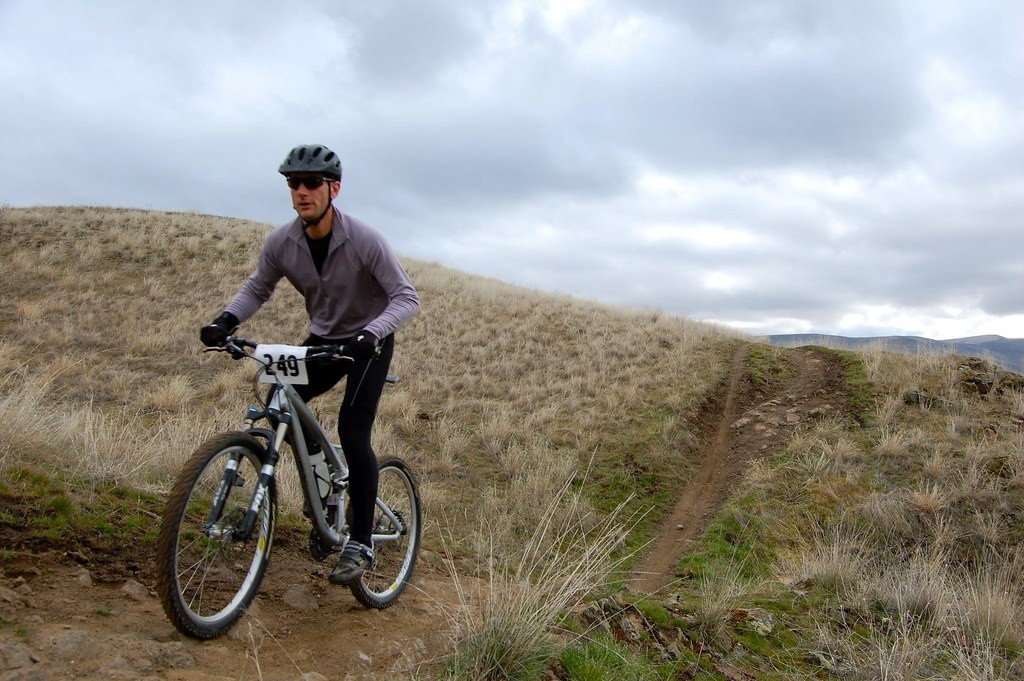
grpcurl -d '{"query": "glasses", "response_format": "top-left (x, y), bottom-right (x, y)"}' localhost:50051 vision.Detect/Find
top-left (285, 176), bottom-right (334, 190)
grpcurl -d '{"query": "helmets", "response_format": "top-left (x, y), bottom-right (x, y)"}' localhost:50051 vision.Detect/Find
top-left (278, 144), bottom-right (342, 177)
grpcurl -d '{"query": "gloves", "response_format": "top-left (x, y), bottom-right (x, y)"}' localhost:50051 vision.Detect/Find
top-left (200, 313), bottom-right (239, 347)
top-left (345, 331), bottom-right (377, 364)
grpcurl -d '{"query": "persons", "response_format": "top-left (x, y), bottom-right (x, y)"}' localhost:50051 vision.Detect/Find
top-left (200, 145), bottom-right (420, 586)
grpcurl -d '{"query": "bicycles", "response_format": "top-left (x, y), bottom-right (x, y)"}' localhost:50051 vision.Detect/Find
top-left (152, 331), bottom-right (424, 640)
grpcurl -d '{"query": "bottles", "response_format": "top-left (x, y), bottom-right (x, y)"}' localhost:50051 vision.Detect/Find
top-left (326, 487), bottom-right (339, 523)
top-left (306, 442), bottom-right (330, 500)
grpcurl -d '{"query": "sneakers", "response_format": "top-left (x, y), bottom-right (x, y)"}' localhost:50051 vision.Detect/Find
top-left (328, 540), bottom-right (374, 582)
top-left (302, 488), bottom-right (325, 516)
